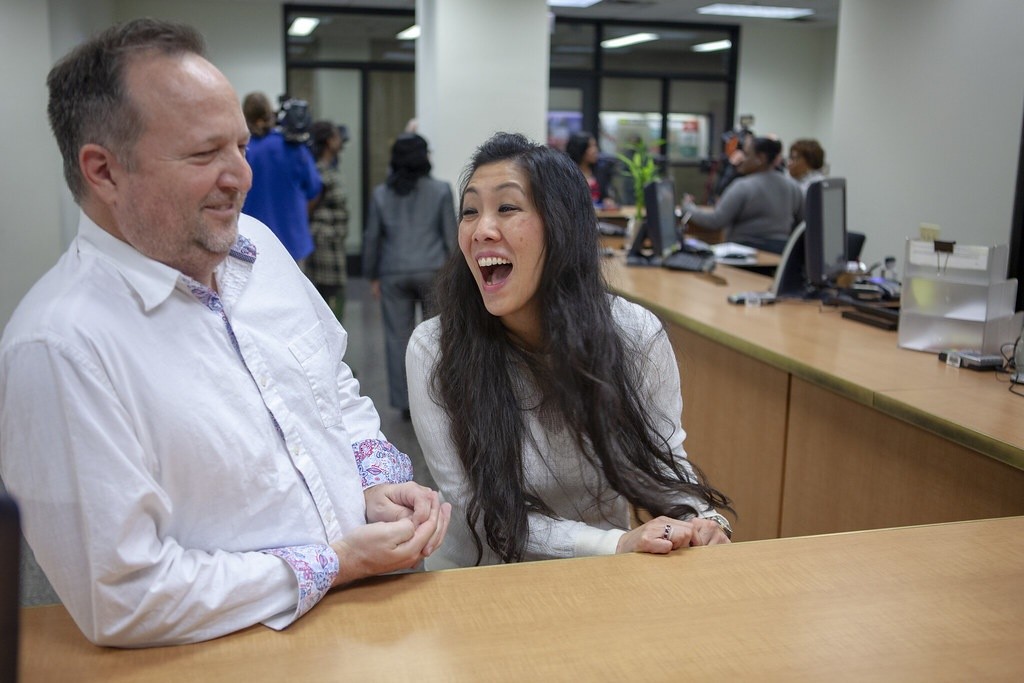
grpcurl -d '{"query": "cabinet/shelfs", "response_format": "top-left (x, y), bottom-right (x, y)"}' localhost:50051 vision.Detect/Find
top-left (17, 515), bottom-right (1023, 683)
top-left (590, 205), bottom-right (1023, 541)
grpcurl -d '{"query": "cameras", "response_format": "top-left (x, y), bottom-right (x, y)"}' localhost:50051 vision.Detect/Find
top-left (276, 95), bottom-right (310, 142)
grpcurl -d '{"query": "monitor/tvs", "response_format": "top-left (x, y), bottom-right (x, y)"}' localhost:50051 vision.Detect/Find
top-left (806, 177), bottom-right (847, 283)
top-left (646, 180), bottom-right (681, 256)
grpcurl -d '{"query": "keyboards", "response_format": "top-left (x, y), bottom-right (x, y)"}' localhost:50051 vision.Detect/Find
top-left (663, 250), bottom-right (711, 273)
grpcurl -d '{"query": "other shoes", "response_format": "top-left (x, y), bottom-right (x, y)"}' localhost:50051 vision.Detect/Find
top-left (399, 406), bottom-right (411, 420)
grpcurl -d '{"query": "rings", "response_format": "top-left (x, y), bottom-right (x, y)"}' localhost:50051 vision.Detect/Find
top-left (663, 524), bottom-right (672, 541)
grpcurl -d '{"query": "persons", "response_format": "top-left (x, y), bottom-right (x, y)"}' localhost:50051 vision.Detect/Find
top-left (0, 17), bottom-right (453, 653)
top-left (559, 128), bottom-right (608, 209)
top-left (303, 114), bottom-right (351, 324)
top-left (402, 131), bottom-right (736, 566)
top-left (783, 136), bottom-right (830, 194)
top-left (682, 135), bottom-right (806, 257)
top-left (362, 130), bottom-right (461, 421)
top-left (239, 90), bottom-right (323, 273)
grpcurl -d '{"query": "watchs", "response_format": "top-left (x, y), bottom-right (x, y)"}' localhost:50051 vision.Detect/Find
top-left (702, 515), bottom-right (733, 540)
